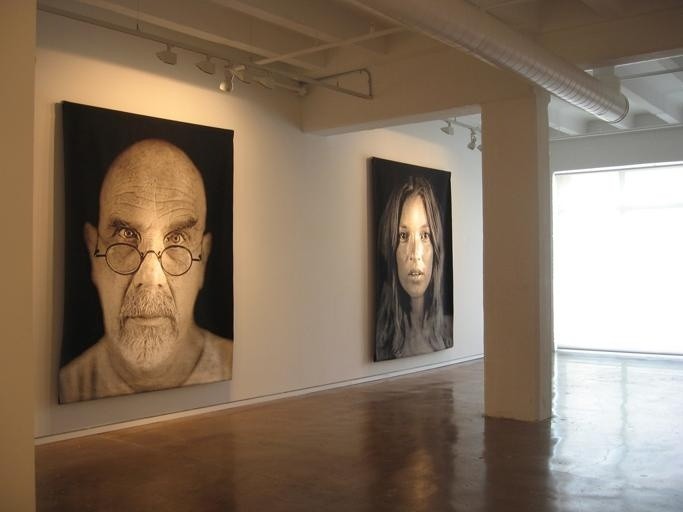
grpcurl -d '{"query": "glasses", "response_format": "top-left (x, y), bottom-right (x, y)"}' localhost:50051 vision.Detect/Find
top-left (91, 233), bottom-right (207, 276)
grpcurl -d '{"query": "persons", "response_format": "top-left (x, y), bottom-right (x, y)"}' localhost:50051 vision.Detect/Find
top-left (374, 171), bottom-right (453, 362)
top-left (57, 138), bottom-right (234, 404)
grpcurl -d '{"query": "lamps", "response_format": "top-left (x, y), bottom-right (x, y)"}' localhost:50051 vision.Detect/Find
top-left (155, 41), bottom-right (307, 100)
top-left (440, 121), bottom-right (481, 151)
